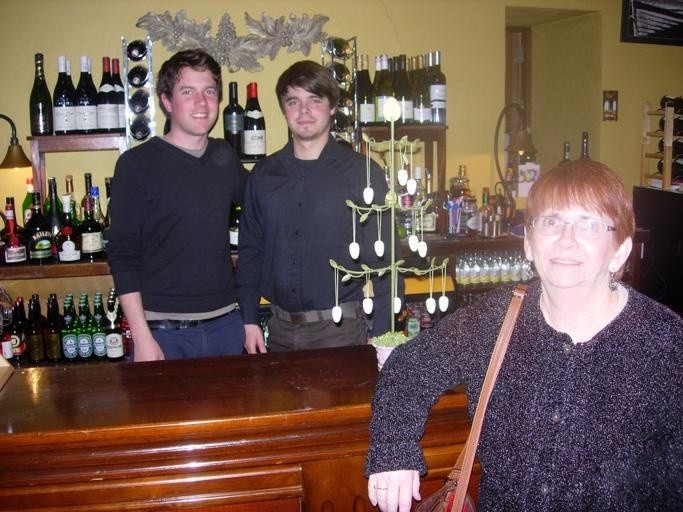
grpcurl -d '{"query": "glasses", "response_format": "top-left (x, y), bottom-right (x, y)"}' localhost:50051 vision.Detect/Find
top-left (525, 214), bottom-right (621, 241)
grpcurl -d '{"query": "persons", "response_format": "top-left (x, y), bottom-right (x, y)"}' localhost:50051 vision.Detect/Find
top-left (235, 60), bottom-right (404, 353)
top-left (365, 160), bottom-right (683, 511)
top-left (108, 50), bottom-right (249, 362)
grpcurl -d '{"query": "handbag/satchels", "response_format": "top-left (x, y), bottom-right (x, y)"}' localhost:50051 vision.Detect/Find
top-left (413, 474), bottom-right (479, 512)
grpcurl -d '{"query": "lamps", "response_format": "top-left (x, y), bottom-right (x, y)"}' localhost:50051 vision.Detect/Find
top-left (0, 113), bottom-right (32, 169)
top-left (495, 104), bottom-right (535, 227)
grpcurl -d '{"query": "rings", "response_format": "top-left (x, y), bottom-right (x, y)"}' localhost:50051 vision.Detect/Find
top-left (375, 485), bottom-right (387, 492)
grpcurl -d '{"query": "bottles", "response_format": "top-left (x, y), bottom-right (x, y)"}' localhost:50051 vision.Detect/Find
top-left (557, 141), bottom-right (574, 166)
top-left (357, 50), bottom-right (446, 127)
top-left (223, 83), bottom-right (244, 159)
top-left (455, 248), bottom-right (533, 288)
top-left (0, 173), bottom-right (118, 264)
top-left (125, 54), bottom-right (151, 143)
top-left (384, 162), bottom-right (439, 235)
top-left (400, 303), bottom-right (436, 337)
top-left (29, 53), bottom-right (53, 136)
top-left (658, 95), bottom-right (683, 179)
top-left (0, 288), bottom-right (132, 363)
top-left (241, 83), bottom-right (268, 160)
top-left (449, 165), bottom-right (516, 240)
top-left (54, 56), bottom-right (126, 135)
top-left (325, 36), bottom-right (356, 151)
top-left (580, 131), bottom-right (591, 159)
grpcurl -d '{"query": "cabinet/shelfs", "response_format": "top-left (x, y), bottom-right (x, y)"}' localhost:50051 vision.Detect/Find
top-left (0, 36), bottom-right (651, 296)
top-left (634, 106), bottom-right (683, 320)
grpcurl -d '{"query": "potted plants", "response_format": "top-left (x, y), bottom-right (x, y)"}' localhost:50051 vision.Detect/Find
top-left (367, 331), bottom-right (410, 372)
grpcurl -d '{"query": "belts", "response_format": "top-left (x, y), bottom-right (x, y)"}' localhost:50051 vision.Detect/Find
top-left (143, 308), bottom-right (237, 331)
top-left (270, 300), bottom-right (367, 325)
